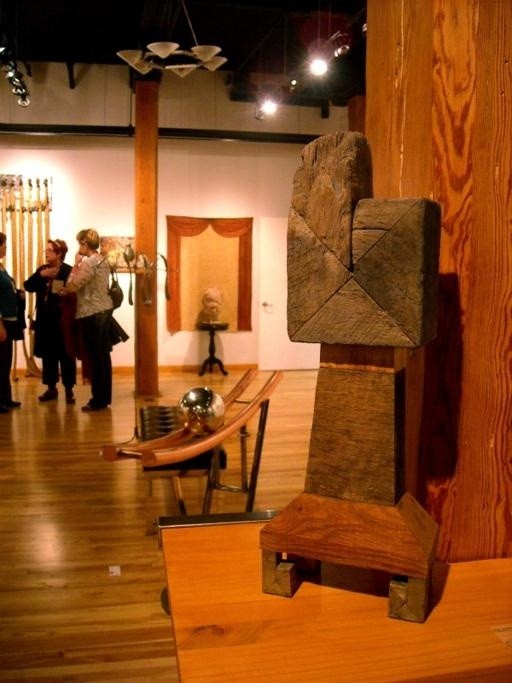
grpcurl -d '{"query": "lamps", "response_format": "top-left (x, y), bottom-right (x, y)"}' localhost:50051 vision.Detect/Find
top-left (258, 95), bottom-right (277, 117)
top-left (308, 58), bottom-right (329, 78)
top-left (116, 0), bottom-right (228, 79)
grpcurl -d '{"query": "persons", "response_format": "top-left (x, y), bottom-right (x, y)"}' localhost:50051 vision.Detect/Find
top-left (23, 239), bottom-right (78, 404)
top-left (66, 229), bottom-right (111, 411)
top-left (0, 232), bottom-right (27, 414)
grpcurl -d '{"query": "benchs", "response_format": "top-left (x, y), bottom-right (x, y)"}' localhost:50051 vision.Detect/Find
top-left (139, 404), bottom-right (227, 479)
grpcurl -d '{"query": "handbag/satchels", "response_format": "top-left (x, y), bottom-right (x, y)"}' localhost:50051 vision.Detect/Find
top-left (108, 280), bottom-right (123, 308)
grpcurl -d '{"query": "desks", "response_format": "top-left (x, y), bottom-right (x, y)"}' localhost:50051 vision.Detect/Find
top-left (198, 322), bottom-right (229, 376)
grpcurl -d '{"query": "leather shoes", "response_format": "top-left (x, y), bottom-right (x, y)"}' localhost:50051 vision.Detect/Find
top-left (0, 402), bottom-right (20, 412)
top-left (65, 388), bottom-right (76, 403)
top-left (39, 388), bottom-right (57, 401)
top-left (82, 401), bottom-right (107, 411)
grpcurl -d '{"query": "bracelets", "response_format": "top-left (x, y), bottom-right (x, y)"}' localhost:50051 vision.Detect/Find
top-left (73, 264), bottom-right (79, 268)
